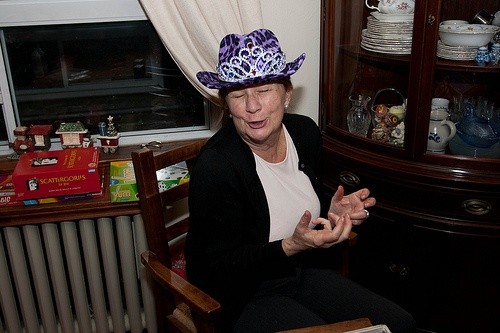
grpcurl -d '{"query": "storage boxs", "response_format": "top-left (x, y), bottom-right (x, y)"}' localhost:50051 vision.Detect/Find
top-left (11, 146), bottom-right (102, 202)
top-left (109, 160), bottom-right (190, 202)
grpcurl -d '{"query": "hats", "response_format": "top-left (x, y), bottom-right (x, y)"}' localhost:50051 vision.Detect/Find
top-left (195, 28), bottom-right (307, 90)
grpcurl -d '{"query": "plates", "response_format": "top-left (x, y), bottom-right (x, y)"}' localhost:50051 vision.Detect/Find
top-left (359, 11), bottom-right (415, 55)
top-left (436, 40), bottom-right (478, 61)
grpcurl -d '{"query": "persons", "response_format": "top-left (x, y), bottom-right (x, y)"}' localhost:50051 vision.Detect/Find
top-left (184, 29), bottom-right (415, 333)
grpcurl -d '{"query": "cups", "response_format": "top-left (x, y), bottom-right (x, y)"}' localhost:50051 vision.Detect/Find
top-left (431, 98), bottom-right (449, 110)
top-left (425, 110), bottom-right (457, 154)
top-left (470, 11), bottom-right (495, 25)
top-left (439, 20), bottom-right (469, 25)
top-left (453, 94), bottom-right (494, 121)
top-left (371, 104), bottom-right (407, 145)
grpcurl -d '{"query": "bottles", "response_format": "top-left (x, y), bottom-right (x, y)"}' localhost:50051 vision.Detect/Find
top-left (346, 94), bottom-right (371, 139)
top-left (475, 47), bottom-right (489, 66)
top-left (489, 43), bottom-right (500, 65)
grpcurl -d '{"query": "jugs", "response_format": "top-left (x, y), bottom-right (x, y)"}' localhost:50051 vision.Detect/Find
top-left (365, 0), bottom-right (416, 14)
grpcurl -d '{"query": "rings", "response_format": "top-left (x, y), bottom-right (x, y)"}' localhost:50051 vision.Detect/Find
top-left (364, 210), bottom-right (369, 218)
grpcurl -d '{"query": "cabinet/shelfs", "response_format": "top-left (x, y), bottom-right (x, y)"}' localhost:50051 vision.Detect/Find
top-left (316, 0), bottom-right (500, 333)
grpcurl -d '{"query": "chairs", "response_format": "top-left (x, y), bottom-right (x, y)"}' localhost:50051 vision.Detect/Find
top-left (131, 138), bottom-right (222, 333)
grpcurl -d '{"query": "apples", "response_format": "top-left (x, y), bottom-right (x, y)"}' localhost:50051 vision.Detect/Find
top-left (389, 105), bottom-right (405, 122)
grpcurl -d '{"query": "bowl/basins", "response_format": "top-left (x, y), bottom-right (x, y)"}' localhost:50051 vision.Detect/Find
top-left (438, 24), bottom-right (500, 46)
top-left (454, 118), bottom-right (500, 147)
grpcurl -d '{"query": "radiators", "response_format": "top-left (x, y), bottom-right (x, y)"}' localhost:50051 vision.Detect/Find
top-left (0, 212), bottom-right (157, 333)
